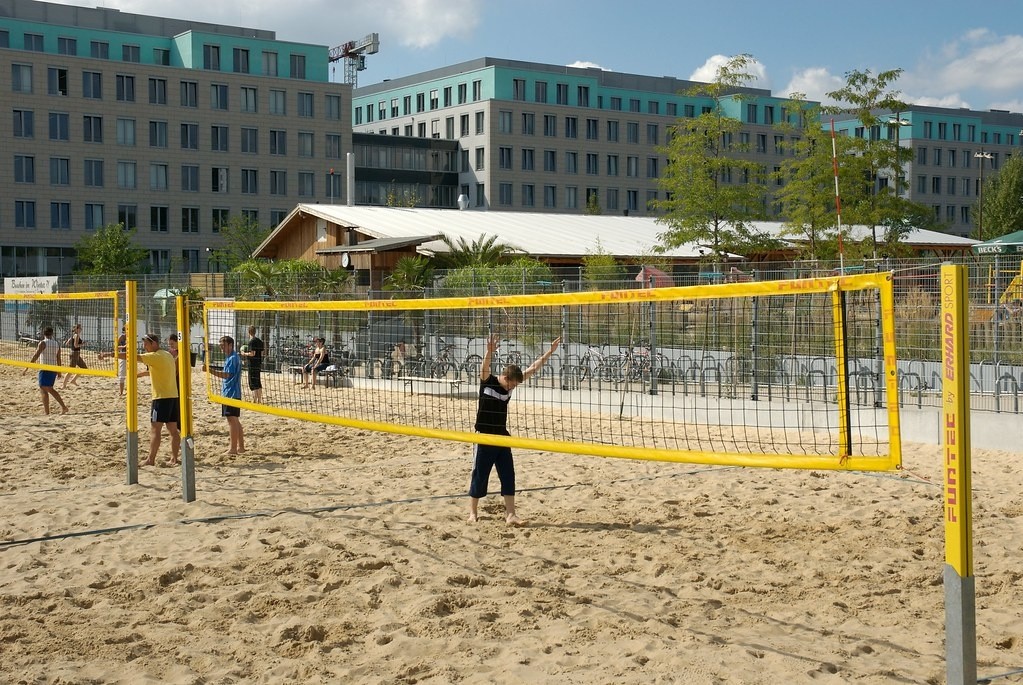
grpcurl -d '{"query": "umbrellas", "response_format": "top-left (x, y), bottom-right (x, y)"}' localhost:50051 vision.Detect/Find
top-left (972, 230), bottom-right (1023, 254)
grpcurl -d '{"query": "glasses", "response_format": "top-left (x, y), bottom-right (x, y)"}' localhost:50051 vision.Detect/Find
top-left (144, 335), bottom-right (154, 341)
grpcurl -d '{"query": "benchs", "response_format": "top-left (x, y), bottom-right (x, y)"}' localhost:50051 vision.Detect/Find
top-left (289, 366), bottom-right (338, 388)
top-left (19, 337), bottom-right (40, 349)
top-left (398, 376), bottom-right (466, 401)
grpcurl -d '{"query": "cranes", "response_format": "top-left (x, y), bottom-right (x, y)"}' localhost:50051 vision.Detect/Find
top-left (328, 32), bottom-right (381, 94)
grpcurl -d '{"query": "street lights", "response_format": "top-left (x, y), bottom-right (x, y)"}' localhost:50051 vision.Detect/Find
top-left (974, 147), bottom-right (995, 244)
top-left (885, 112), bottom-right (915, 217)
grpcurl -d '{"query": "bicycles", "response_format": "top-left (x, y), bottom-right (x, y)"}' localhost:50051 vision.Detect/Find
top-left (261, 335), bottom-right (358, 374)
top-left (568, 343), bottom-right (684, 383)
top-left (17, 329), bottom-right (169, 353)
top-left (380, 330), bottom-right (532, 378)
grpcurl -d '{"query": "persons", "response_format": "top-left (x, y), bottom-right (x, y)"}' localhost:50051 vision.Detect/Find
top-left (113, 326), bottom-right (126, 396)
top-left (168, 333), bottom-right (181, 431)
top-left (202, 336), bottom-right (245, 455)
top-left (994, 298), bottom-right (1023, 345)
top-left (241, 326), bottom-right (264, 403)
top-left (20, 332), bottom-right (41, 347)
top-left (467, 333), bottom-right (561, 525)
top-left (61, 324), bottom-right (88, 390)
top-left (21, 326), bottom-right (69, 415)
top-left (98, 334), bottom-right (180, 466)
top-left (302, 338), bottom-right (330, 390)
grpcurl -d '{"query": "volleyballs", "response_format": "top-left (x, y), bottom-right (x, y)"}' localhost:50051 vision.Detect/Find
top-left (240, 345), bottom-right (249, 353)
